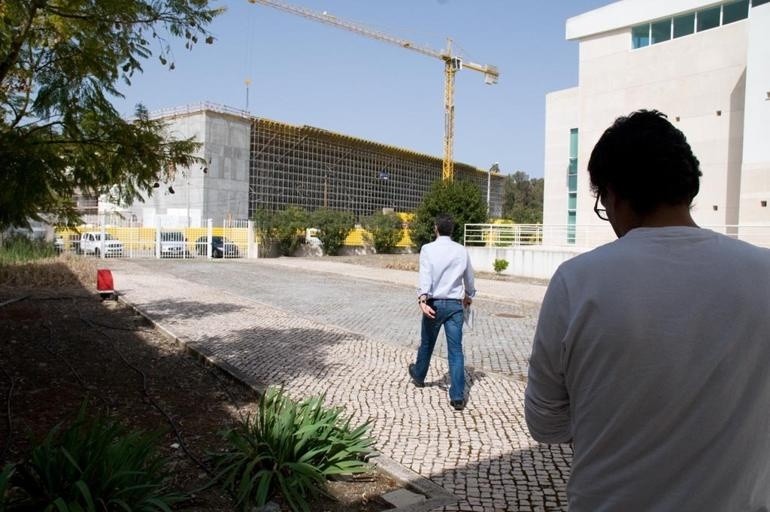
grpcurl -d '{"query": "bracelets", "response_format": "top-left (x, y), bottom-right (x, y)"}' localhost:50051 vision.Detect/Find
top-left (418, 299), bottom-right (426, 305)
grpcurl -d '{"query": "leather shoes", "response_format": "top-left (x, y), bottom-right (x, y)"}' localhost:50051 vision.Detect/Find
top-left (408, 363), bottom-right (425, 388)
top-left (450, 399), bottom-right (465, 410)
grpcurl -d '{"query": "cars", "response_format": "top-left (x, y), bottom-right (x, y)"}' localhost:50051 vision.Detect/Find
top-left (55, 234), bottom-right (79, 252)
top-left (196, 235), bottom-right (240, 258)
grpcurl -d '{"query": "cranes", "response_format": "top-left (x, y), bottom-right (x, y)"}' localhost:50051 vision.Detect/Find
top-left (251, 0), bottom-right (499, 188)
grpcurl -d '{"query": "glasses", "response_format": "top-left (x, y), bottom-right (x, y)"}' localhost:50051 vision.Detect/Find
top-left (593, 188), bottom-right (608, 222)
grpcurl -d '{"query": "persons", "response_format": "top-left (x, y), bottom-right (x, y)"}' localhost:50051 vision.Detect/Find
top-left (408, 218), bottom-right (476, 410)
top-left (524, 109), bottom-right (770, 512)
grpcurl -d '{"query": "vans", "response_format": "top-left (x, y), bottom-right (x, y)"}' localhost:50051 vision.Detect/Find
top-left (155, 231), bottom-right (190, 257)
top-left (81, 232), bottom-right (122, 256)
